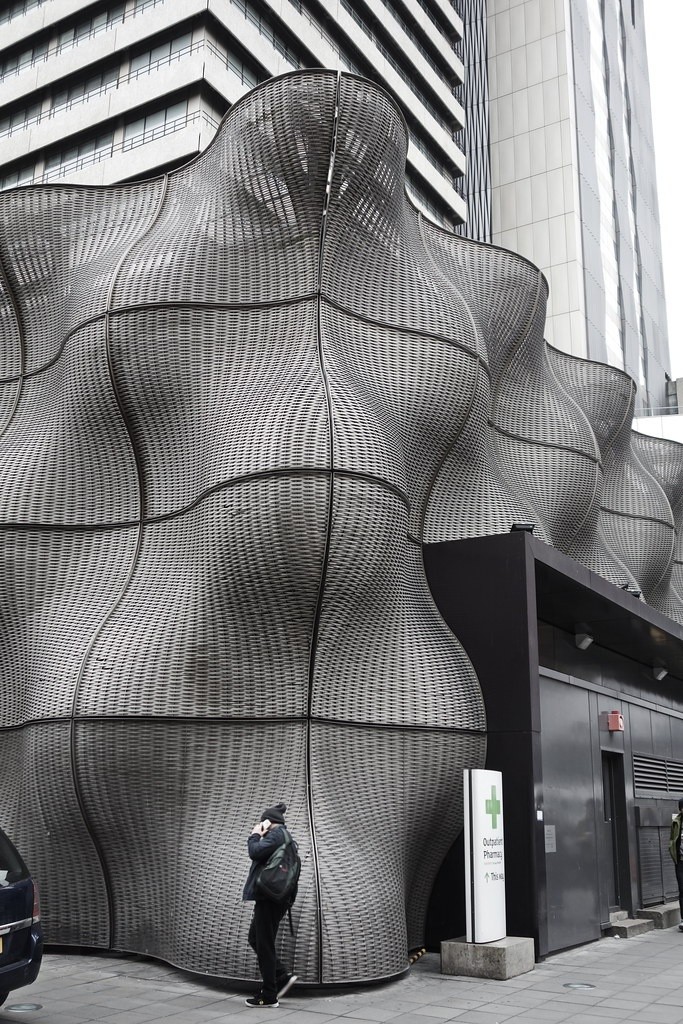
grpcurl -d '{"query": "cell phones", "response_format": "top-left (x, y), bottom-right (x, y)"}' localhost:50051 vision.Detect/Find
top-left (262, 818), bottom-right (272, 832)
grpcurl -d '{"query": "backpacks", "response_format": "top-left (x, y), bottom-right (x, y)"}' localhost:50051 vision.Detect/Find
top-left (255, 827), bottom-right (301, 907)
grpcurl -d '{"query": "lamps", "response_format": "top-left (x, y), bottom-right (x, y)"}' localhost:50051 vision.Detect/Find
top-left (653, 667), bottom-right (668, 681)
top-left (627, 590), bottom-right (642, 599)
top-left (510, 523), bottom-right (536, 535)
top-left (575, 633), bottom-right (594, 650)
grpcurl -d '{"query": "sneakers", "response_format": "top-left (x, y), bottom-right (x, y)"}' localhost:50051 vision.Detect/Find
top-left (274, 972), bottom-right (297, 999)
top-left (245, 991), bottom-right (279, 1008)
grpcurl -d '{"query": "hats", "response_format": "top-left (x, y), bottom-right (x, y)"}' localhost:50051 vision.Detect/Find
top-left (679, 798), bottom-right (683, 810)
top-left (260, 802), bottom-right (287, 825)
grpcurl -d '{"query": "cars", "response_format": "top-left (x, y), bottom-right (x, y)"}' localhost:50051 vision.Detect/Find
top-left (0, 828), bottom-right (44, 1007)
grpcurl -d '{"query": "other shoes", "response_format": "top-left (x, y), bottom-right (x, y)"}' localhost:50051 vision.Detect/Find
top-left (679, 919), bottom-right (683, 930)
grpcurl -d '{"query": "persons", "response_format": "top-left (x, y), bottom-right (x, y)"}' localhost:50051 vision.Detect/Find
top-left (242, 802), bottom-right (302, 1008)
top-left (669, 798), bottom-right (683, 932)
